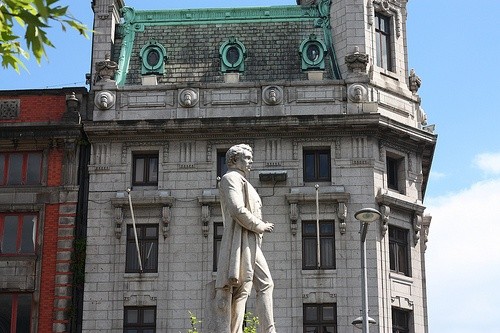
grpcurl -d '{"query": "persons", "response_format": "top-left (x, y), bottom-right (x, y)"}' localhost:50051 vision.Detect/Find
top-left (215, 144), bottom-right (275, 333)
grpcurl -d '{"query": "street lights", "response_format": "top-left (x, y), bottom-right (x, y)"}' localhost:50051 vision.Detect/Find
top-left (351, 207), bottom-right (382, 333)
